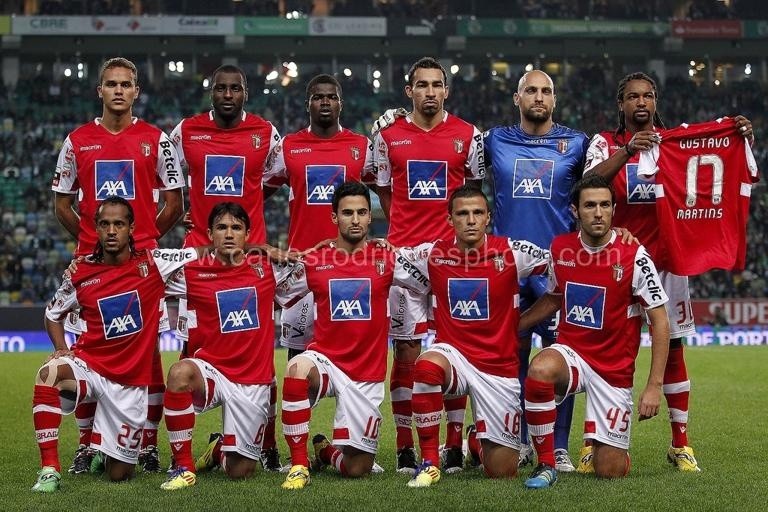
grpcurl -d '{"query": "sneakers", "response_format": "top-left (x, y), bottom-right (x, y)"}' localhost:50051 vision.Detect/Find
top-left (30, 466), bottom-right (61, 495)
top-left (434, 426), bottom-right (595, 473)
top-left (263, 434), bottom-right (418, 474)
top-left (159, 465), bottom-right (199, 491)
top-left (68, 432), bottom-right (226, 476)
top-left (405, 458), bottom-right (441, 487)
top-left (524, 463), bottom-right (558, 490)
top-left (281, 464), bottom-right (312, 489)
top-left (667, 440), bottom-right (700, 472)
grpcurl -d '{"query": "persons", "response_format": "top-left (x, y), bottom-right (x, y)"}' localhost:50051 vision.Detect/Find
top-left (2, 52), bottom-right (767, 328)
top-left (26, 57), bottom-right (752, 493)
